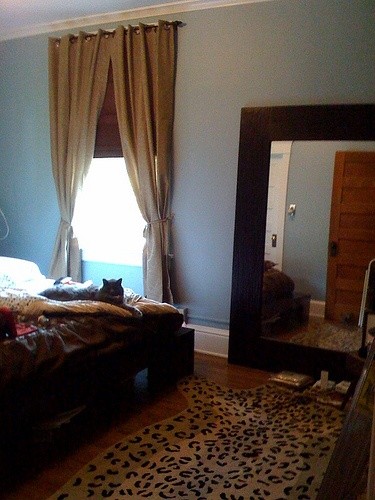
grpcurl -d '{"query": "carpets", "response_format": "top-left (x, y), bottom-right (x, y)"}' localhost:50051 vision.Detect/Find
top-left (285, 323), bottom-right (370, 353)
top-left (50, 373), bottom-right (348, 499)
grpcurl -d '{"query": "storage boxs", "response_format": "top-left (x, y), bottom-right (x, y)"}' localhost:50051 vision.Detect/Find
top-left (29, 405), bottom-right (88, 465)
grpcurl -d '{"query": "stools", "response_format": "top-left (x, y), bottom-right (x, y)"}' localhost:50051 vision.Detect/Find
top-left (291, 291), bottom-right (310, 329)
top-left (169, 326), bottom-right (196, 375)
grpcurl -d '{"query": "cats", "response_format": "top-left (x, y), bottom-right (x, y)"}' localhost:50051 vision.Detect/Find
top-left (263, 258), bottom-right (278, 273)
top-left (38, 277), bottom-right (144, 318)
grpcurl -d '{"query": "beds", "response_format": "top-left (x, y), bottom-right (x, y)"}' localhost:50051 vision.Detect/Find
top-left (1, 255), bottom-right (195, 479)
top-left (261, 259), bottom-right (295, 336)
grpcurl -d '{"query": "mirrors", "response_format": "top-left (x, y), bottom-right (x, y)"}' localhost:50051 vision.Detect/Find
top-left (226, 102), bottom-right (375, 382)
